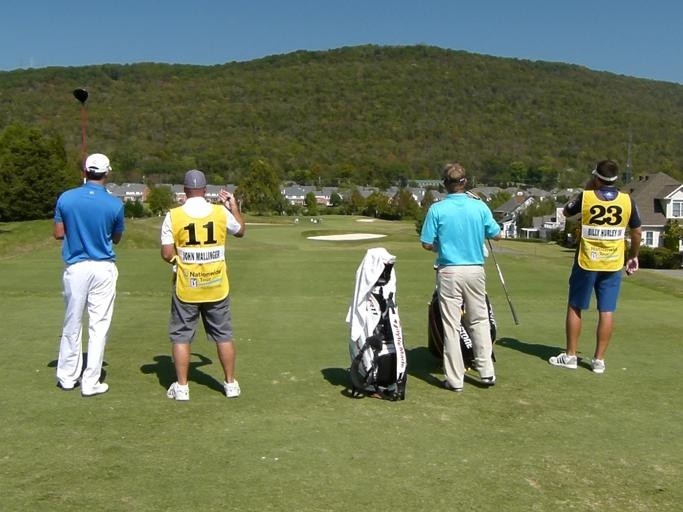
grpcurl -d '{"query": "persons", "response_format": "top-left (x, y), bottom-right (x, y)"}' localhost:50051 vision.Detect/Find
top-left (549, 160), bottom-right (642, 374)
top-left (419, 161), bottom-right (502, 393)
top-left (54, 155), bottom-right (126, 398)
top-left (161, 169), bottom-right (246, 401)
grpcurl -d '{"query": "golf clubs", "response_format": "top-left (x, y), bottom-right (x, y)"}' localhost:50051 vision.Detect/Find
top-left (73, 88), bottom-right (88, 181)
top-left (466, 190), bottom-right (518, 325)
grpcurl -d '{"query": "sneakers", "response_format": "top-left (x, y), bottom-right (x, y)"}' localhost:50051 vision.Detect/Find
top-left (167, 381), bottom-right (191, 401)
top-left (589, 355), bottom-right (607, 374)
top-left (80, 380), bottom-right (110, 395)
top-left (548, 351), bottom-right (579, 370)
top-left (479, 374), bottom-right (496, 388)
top-left (226, 381), bottom-right (242, 400)
top-left (438, 377), bottom-right (463, 394)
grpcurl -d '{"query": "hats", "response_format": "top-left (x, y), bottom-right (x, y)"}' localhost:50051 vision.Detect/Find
top-left (590, 160), bottom-right (621, 183)
top-left (183, 169), bottom-right (207, 191)
top-left (441, 166), bottom-right (469, 185)
top-left (84, 152), bottom-right (111, 173)
